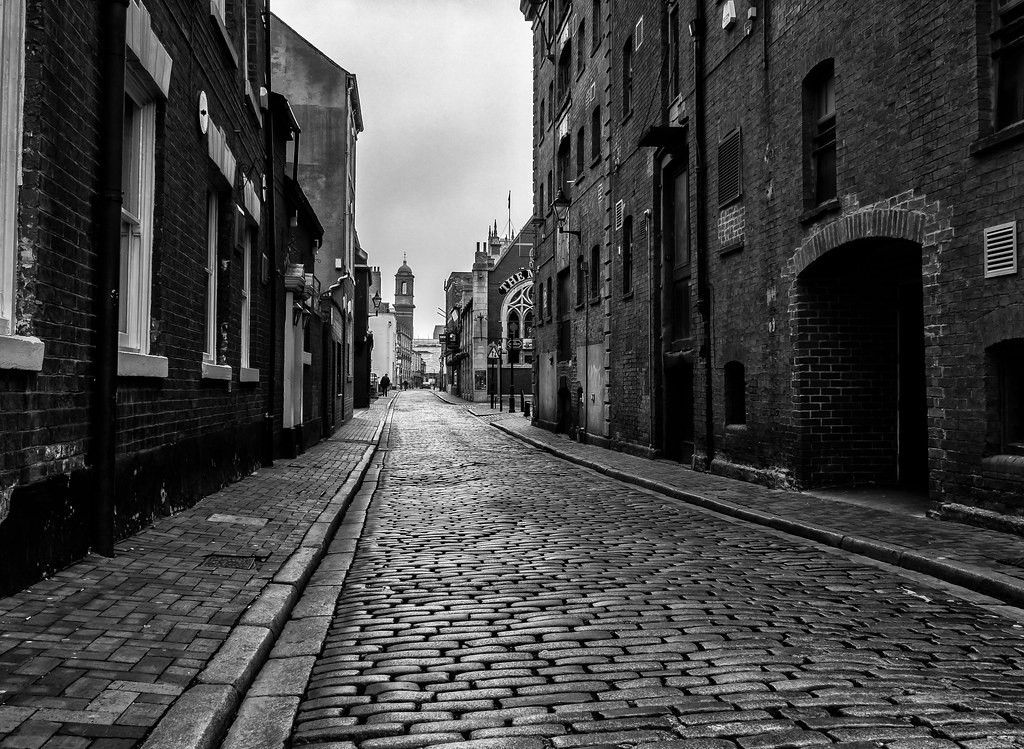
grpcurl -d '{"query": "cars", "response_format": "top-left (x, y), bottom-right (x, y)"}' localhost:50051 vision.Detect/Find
top-left (420, 382), bottom-right (431, 389)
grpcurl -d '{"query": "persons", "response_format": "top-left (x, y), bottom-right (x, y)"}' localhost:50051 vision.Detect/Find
top-left (380, 374), bottom-right (390, 397)
top-left (403, 379), bottom-right (422, 391)
